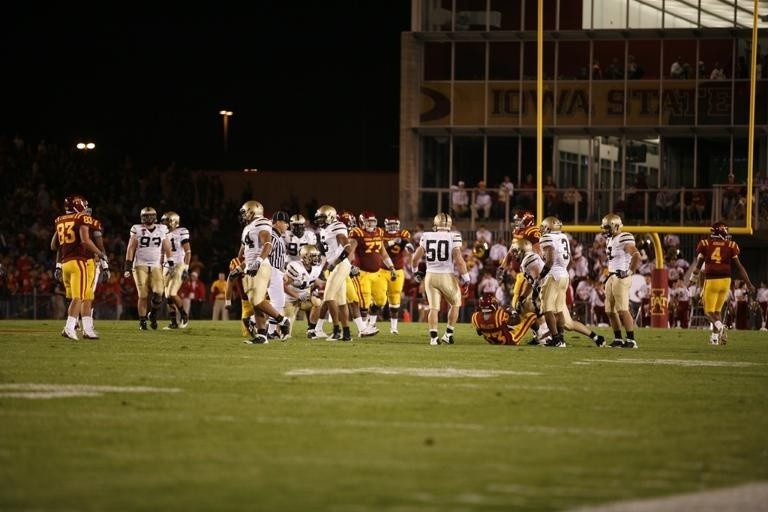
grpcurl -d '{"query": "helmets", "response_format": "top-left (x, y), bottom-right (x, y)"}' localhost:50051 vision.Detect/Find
top-left (63, 194), bottom-right (88, 214)
top-left (140, 206), bottom-right (181, 230)
top-left (601, 214), bottom-right (623, 238)
top-left (337, 209), bottom-right (401, 235)
top-left (479, 292), bottom-right (498, 312)
top-left (510, 209), bottom-right (562, 264)
top-left (239, 200), bottom-right (337, 229)
top-left (299, 244), bottom-right (320, 266)
top-left (433, 213), bottom-right (452, 231)
top-left (710, 223), bottom-right (728, 241)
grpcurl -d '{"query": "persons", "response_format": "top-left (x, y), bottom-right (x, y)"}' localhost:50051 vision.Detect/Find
top-left (51, 196), bottom-right (112, 342)
top-left (451, 177), bottom-right (768, 224)
top-left (123, 207), bottom-right (192, 330)
top-left (2, 128), bottom-right (205, 322)
top-left (226, 201), bottom-right (767, 349)
top-left (208, 272), bottom-right (231, 320)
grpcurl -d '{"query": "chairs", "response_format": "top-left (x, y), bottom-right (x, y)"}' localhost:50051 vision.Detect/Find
top-left (563, 254), bottom-right (719, 331)
top-left (390, 243), bottom-right (501, 327)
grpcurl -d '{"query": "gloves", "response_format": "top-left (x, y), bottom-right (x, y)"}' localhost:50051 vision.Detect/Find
top-left (390, 268), bottom-right (396, 280)
top-left (228, 261), bottom-right (260, 281)
top-left (462, 281), bottom-right (470, 298)
top-left (350, 266), bottom-right (360, 276)
top-left (100, 252), bottom-right (112, 284)
top-left (298, 292), bottom-right (308, 301)
top-left (54, 267), bottom-right (62, 281)
top-left (616, 269), bottom-right (628, 278)
top-left (166, 268), bottom-right (188, 281)
top-left (413, 270), bottom-right (424, 283)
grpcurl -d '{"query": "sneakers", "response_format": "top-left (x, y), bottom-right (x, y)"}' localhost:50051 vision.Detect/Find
top-left (61, 320), bottom-right (99, 340)
top-left (243, 317), bottom-right (454, 346)
top-left (710, 325), bottom-right (728, 345)
top-left (527, 335), bottom-right (638, 348)
top-left (139, 311), bottom-right (189, 330)
